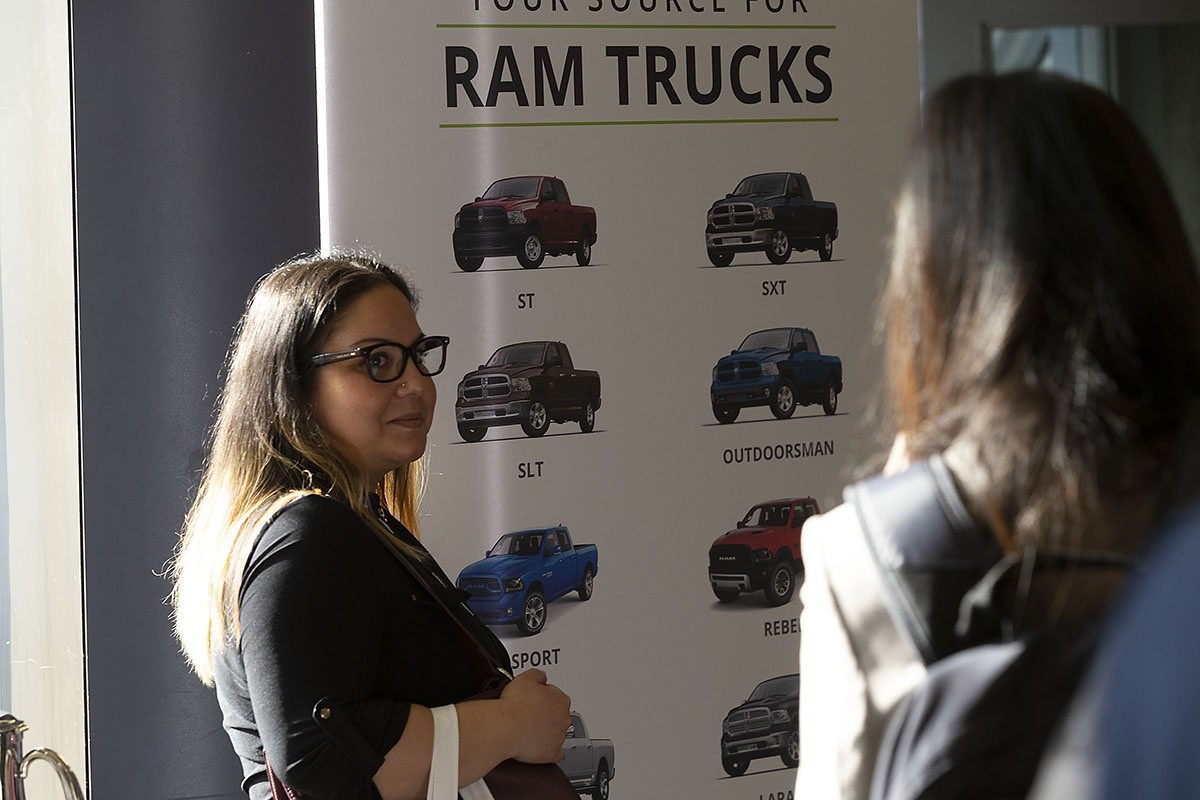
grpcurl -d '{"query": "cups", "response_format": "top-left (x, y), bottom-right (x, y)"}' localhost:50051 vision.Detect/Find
top-left (0, 711), bottom-right (83, 800)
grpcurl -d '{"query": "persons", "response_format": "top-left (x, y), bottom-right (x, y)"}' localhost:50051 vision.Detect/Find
top-left (792, 70), bottom-right (1200, 800)
top-left (161, 246), bottom-right (572, 800)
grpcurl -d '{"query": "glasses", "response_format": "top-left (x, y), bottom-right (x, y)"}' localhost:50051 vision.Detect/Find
top-left (309, 336), bottom-right (450, 383)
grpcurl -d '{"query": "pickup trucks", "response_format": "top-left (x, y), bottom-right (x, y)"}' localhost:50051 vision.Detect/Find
top-left (720, 672), bottom-right (800, 778)
top-left (454, 523), bottom-right (599, 637)
top-left (452, 174), bottom-right (598, 273)
top-left (454, 340), bottom-right (602, 444)
top-left (704, 172), bottom-right (839, 269)
top-left (554, 710), bottom-right (617, 800)
top-left (708, 326), bottom-right (843, 424)
top-left (706, 496), bottom-right (822, 608)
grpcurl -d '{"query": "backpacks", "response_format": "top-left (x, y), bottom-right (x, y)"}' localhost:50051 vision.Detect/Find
top-left (850, 446), bottom-right (1142, 800)
